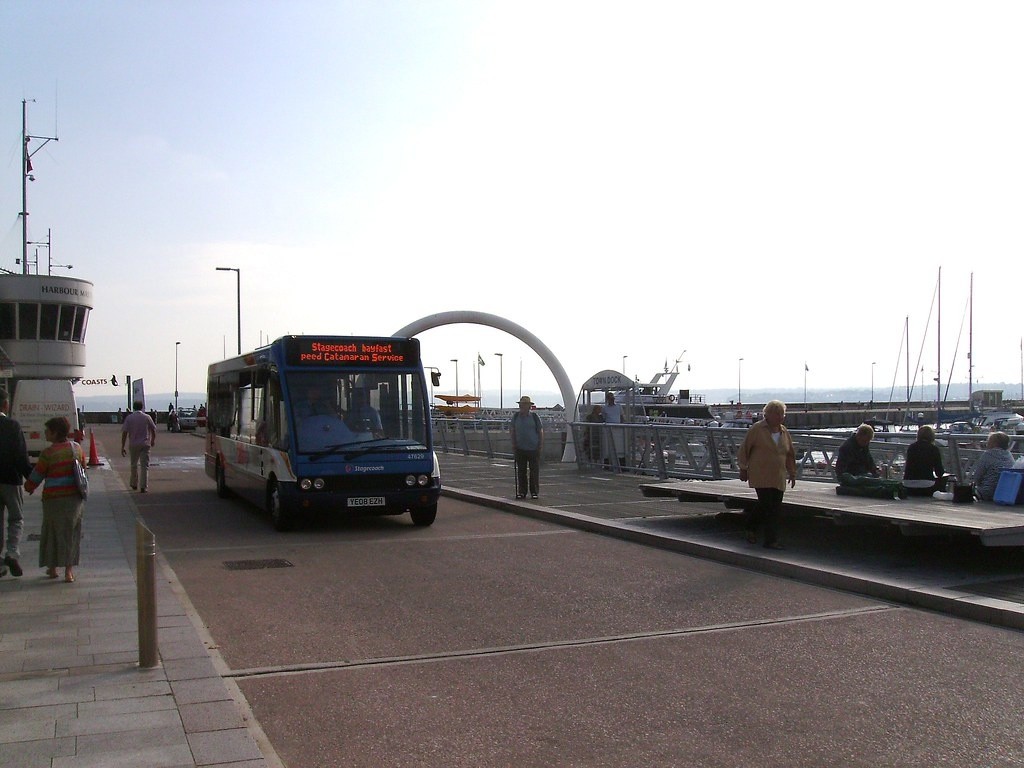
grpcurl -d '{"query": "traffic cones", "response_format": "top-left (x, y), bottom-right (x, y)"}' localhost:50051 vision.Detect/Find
top-left (87, 431), bottom-right (105, 465)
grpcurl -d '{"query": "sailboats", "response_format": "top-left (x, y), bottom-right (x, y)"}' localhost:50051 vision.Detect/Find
top-left (833, 265), bottom-right (1024, 462)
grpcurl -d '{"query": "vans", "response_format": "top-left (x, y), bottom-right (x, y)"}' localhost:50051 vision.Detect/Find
top-left (10, 379), bottom-right (82, 469)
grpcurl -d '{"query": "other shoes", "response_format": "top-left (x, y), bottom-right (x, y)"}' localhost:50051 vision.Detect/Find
top-left (46, 569), bottom-right (60, 578)
top-left (130, 484), bottom-right (138, 490)
top-left (517, 493), bottom-right (526, 499)
top-left (141, 487), bottom-right (148, 492)
top-left (763, 541), bottom-right (784, 550)
top-left (531, 494), bottom-right (538, 499)
top-left (65, 572), bottom-right (74, 582)
top-left (744, 527), bottom-right (757, 544)
top-left (5, 555), bottom-right (22, 576)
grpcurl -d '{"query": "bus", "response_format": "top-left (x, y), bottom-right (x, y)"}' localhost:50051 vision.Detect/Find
top-left (205, 334), bottom-right (441, 532)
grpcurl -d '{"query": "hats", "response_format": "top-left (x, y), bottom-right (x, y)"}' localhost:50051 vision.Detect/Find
top-left (516, 396), bottom-right (534, 405)
top-left (606, 393), bottom-right (615, 399)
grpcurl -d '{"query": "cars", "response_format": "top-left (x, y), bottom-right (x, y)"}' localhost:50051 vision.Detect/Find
top-left (167, 408), bottom-right (199, 430)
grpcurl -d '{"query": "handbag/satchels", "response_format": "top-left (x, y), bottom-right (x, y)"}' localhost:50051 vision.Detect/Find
top-left (70, 439), bottom-right (90, 501)
top-left (952, 477), bottom-right (975, 503)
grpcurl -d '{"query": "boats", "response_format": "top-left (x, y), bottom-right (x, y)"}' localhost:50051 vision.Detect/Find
top-left (594, 350), bottom-right (716, 426)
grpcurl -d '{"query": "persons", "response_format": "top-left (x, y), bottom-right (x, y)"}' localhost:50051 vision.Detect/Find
top-left (898, 426), bottom-right (954, 500)
top-left (169, 402), bottom-right (181, 432)
top-left (509, 396), bottom-right (545, 499)
top-left (122, 401), bottom-right (156, 493)
top-left (292, 383), bottom-right (331, 426)
top-left (193, 404), bottom-right (206, 416)
top-left (24, 417), bottom-right (89, 583)
top-left (835, 425), bottom-right (882, 487)
top-left (77, 408), bottom-right (86, 441)
top-left (737, 400), bottom-right (796, 550)
top-left (117, 407), bottom-right (131, 424)
top-left (681, 417), bottom-right (720, 426)
top-left (0, 389), bottom-right (33, 578)
top-left (973, 432), bottom-right (1016, 502)
top-left (149, 409), bottom-right (158, 426)
top-left (583, 392), bottom-right (627, 471)
top-left (343, 388), bottom-right (384, 439)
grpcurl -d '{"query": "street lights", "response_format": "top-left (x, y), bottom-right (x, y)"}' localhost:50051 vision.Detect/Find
top-left (494, 353), bottom-right (502, 409)
top-left (623, 356), bottom-right (628, 374)
top-left (216, 268), bottom-right (241, 354)
top-left (451, 360), bottom-right (458, 407)
top-left (49, 264), bottom-right (73, 276)
top-left (739, 359), bottom-right (744, 410)
top-left (872, 362), bottom-right (876, 402)
top-left (176, 342), bottom-right (181, 413)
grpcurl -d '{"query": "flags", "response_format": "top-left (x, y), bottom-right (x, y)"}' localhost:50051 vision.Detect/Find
top-left (478, 354), bottom-right (486, 366)
